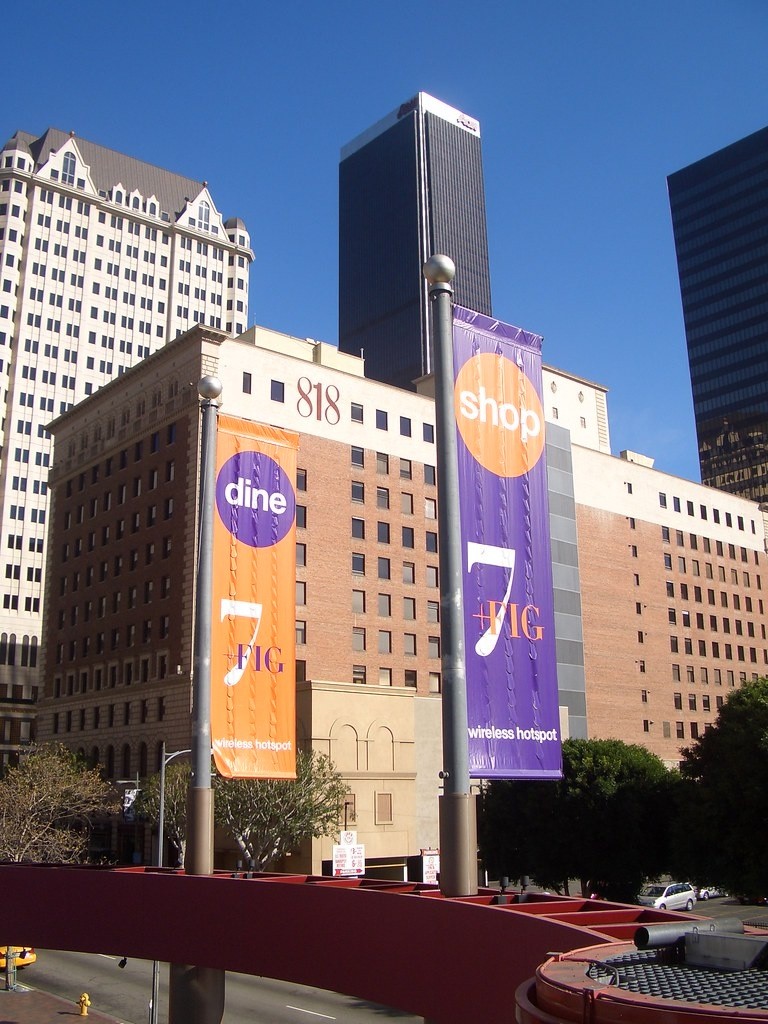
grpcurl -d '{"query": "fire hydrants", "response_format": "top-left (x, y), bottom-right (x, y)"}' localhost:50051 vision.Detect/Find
top-left (79, 993), bottom-right (91, 1015)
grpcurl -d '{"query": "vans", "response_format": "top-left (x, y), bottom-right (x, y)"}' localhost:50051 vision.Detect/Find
top-left (637, 881), bottom-right (697, 911)
top-left (0, 946), bottom-right (36, 970)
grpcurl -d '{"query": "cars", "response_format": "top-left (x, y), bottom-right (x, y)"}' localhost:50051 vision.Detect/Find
top-left (694, 886), bottom-right (729, 900)
